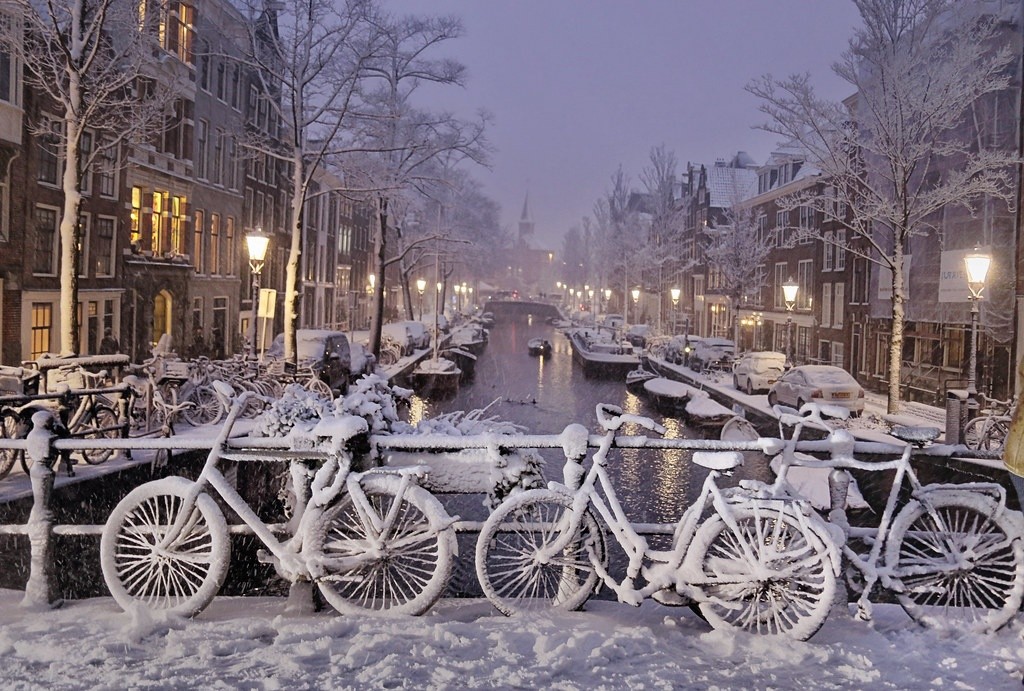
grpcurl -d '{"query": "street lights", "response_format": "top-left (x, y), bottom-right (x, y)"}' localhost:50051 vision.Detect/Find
top-left (244, 222), bottom-right (271, 379)
top-left (781, 275), bottom-right (800, 373)
top-left (605, 288), bottom-right (611, 316)
top-left (631, 287), bottom-right (640, 326)
top-left (417, 277), bottom-right (427, 322)
top-left (961, 240), bottom-right (992, 447)
top-left (670, 283), bottom-right (682, 336)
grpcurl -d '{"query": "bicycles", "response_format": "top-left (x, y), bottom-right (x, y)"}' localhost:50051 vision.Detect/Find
top-left (0, 351), bottom-right (336, 482)
top-left (673, 403), bottom-right (1024, 639)
top-left (359, 334), bottom-right (407, 369)
top-left (475, 402), bottom-right (842, 642)
top-left (99, 379), bottom-right (463, 621)
top-left (962, 392), bottom-right (1017, 454)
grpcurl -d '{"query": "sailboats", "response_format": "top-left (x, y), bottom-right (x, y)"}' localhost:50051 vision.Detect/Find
top-left (408, 205), bottom-right (462, 395)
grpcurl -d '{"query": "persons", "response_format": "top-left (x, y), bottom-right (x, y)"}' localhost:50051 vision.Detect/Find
top-left (211, 328), bottom-right (224, 360)
top-left (99, 327), bottom-right (119, 354)
top-left (539, 292), bottom-right (546, 298)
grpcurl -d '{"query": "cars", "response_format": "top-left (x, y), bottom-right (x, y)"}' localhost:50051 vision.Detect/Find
top-left (733, 352), bottom-right (796, 395)
top-left (688, 337), bottom-right (734, 374)
top-left (768, 365), bottom-right (865, 420)
top-left (603, 313), bottom-right (702, 365)
top-left (380, 320), bottom-right (431, 357)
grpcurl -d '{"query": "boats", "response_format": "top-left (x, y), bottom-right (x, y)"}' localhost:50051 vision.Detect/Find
top-left (551, 318), bottom-right (571, 328)
top-left (643, 378), bottom-right (710, 410)
top-left (770, 451), bottom-right (870, 517)
top-left (685, 391), bottom-right (738, 428)
top-left (528, 337), bottom-right (551, 357)
top-left (626, 364), bottom-right (659, 394)
top-left (566, 327), bottom-right (642, 375)
top-left (431, 311), bottom-right (496, 382)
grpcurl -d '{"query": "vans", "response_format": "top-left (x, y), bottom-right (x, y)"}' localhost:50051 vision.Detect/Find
top-left (489, 289), bottom-right (520, 301)
top-left (262, 329), bottom-right (351, 396)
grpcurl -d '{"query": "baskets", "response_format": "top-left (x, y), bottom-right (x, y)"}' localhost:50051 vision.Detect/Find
top-left (165, 362), bottom-right (188, 378)
top-left (285, 361), bottom-right (297, 374)
top-left (270, 361), bottom-right (284, 376)
